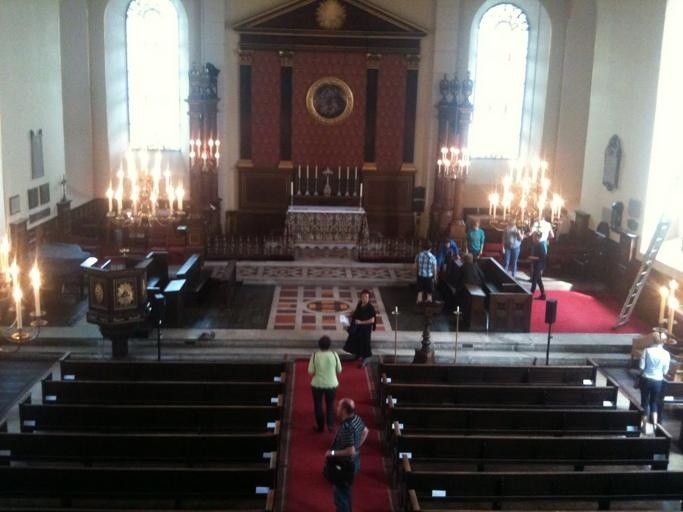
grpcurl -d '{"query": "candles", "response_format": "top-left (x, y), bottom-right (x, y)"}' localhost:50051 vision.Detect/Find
top-left (289, 182), bottom-right (294, 195)
top-left (33, 288), bottom-right (40, 315)
top-left (359, 183), bottom-right (363, 195)
top-left (15, 300), bottom-right (21, 330)
top-left (297, 162), bottom-right (359, 181)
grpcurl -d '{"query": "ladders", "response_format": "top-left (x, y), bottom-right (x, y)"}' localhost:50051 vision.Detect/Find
top-left (611, 212), bottom-right (672, 330)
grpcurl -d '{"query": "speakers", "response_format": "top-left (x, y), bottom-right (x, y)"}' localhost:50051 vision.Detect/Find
top-left (545, 299), bottom-right (557, 323)
top-left (153, 293), bottom-right (165, 326)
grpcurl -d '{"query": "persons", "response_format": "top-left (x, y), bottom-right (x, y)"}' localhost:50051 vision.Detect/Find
top-left (638, 330), bottom-right (671, 432)
top-left (307, 335), bottom-right (344, 434)
top-left (413, 212), bottom-right (556, 306)
top-left (342, 288), bottom-right (377, 369)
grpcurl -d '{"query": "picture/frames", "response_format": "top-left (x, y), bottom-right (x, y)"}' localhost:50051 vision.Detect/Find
top-left (304, 77), bottom-right (355, 126)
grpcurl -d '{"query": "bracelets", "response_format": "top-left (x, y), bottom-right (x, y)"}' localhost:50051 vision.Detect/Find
top-left (331, 449), bottom-right (335, 456)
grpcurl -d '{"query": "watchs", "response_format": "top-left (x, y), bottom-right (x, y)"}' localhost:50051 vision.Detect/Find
top-left (321, 397), bottom-right (370, 512)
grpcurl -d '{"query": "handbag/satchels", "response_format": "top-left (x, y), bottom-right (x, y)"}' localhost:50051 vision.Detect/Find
top-left (634, 375), bottom-right (641, 389)
top-left (328, 457), bottom-right (355, 488)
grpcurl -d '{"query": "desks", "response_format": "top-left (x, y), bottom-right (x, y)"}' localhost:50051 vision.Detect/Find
top-left (286, 205), bottom-right (365, 245)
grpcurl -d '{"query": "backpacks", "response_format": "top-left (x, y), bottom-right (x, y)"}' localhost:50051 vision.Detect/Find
top-left (348, 308), bottom-right (368, 336)
